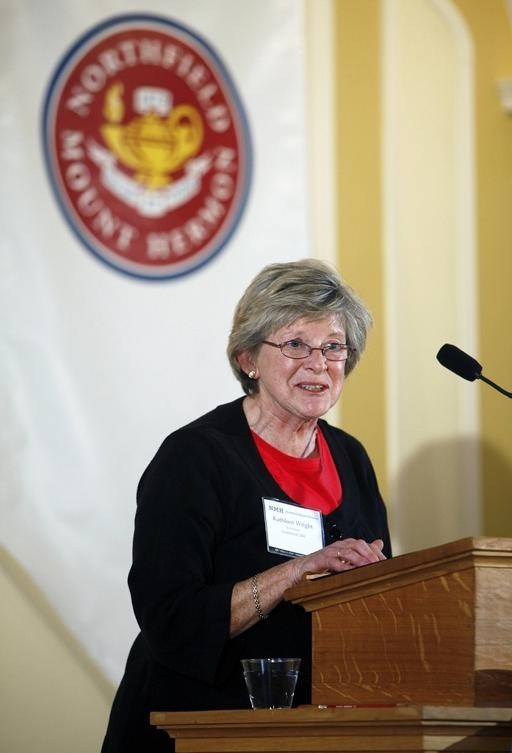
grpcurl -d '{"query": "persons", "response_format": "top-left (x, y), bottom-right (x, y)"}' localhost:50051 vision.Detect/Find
top-left (100, 258), bottom-right (392, 753)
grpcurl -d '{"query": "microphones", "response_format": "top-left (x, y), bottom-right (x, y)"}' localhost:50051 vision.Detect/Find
top-left (436, 344), bottom-right (512, 401)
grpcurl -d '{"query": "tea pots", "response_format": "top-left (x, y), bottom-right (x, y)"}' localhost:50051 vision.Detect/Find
top-left (101, 81), bottom-right (205, 194)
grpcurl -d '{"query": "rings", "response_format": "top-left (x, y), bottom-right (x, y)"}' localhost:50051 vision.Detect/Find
top-left (336, 547), bottom-right (342, 558)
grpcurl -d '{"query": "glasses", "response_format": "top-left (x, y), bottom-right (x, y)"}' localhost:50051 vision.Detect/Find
top-left (262, 340), bottom-right (353, 361)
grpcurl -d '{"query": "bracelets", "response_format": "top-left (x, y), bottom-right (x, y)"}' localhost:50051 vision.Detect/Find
top-left (252, 574), bottom-right (270, 622)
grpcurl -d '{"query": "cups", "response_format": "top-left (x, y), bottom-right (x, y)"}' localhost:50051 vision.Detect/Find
top-left (238, 656), bottom-right (303, 709)
